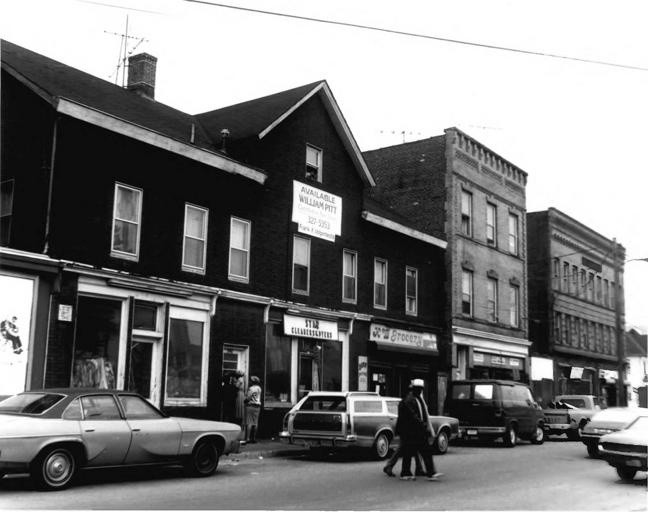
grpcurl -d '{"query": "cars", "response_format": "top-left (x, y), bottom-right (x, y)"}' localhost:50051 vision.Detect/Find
top-left (277, 391), bottom-right (460, 461)
top-left (0, 387), bottom-right (243, 489)
top-left (580, 405), bottom-right (648, 481)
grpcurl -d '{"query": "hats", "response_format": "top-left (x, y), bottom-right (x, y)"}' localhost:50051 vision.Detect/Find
top-left (408, 378), bottom-right (425, 388)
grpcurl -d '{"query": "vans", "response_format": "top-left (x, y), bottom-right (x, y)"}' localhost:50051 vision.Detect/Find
top-left (442, 378), bottom-right (546, 448)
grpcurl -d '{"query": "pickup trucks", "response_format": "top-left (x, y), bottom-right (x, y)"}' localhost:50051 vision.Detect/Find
top-left (543, 394), bottom-right (603, 442)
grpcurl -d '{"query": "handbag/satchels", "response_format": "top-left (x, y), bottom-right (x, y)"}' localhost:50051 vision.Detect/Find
top-left (248, 394), bottom-right (258, 405)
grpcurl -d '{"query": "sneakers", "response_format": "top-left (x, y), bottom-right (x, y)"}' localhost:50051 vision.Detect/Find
top-left (383, 466), bottom-right (445, 480)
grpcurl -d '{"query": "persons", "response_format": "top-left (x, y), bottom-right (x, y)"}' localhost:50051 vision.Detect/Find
top-left (219, 372), bottom-right (240, 423)
top-left (398, 378), bottom-right (443, 480)
top-left (381, 383), bottom-right (427, 477)
top-left (243, 376), bottom-right (262, 444)
